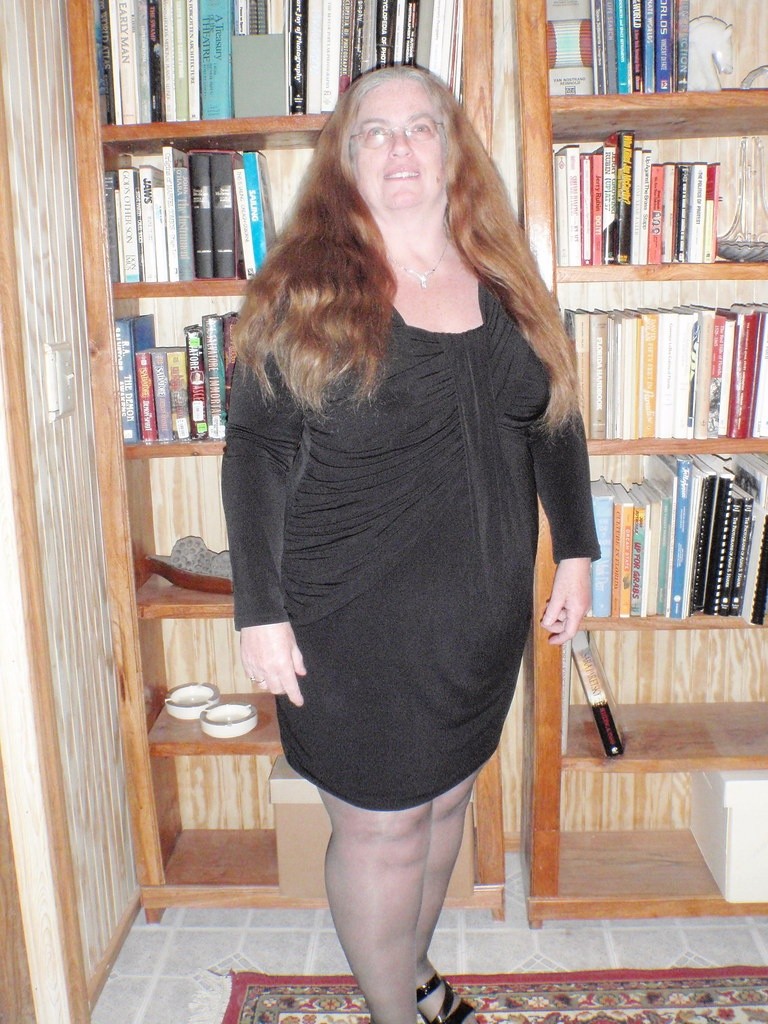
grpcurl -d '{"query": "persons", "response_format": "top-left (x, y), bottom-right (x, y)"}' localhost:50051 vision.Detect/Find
top-left (217, 60), bottom-right (607, 1024)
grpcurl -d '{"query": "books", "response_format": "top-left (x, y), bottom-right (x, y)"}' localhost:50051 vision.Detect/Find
top-left (570, 627), bottom-right (625, 757)
top-left (550, 127), bottom-right (721, 268)
top-left (589, 454), bottom-right (768, 628)
top-left (563, 302), bottom-right (768, 442)
top-left (105, 142), bottom-right (315, 286)
top-left (589, 0), bottom-right (690, 97)
top-left (114, 310), bottom-right (239, 447)
top-left (91, 0), bottom-right (466, 130)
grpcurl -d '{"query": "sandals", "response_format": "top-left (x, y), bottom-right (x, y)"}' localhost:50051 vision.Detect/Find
top-left (416, 972), bottom-right (476, 1024)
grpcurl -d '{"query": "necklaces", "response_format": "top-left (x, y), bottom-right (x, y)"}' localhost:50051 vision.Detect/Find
top-left (383, 219), bottom-right (450, 290)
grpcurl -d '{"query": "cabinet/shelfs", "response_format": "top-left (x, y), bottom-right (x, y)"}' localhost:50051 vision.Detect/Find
top-left (513, 0), bottom-right (768, 927)
top-left (66, 0), bottom-right (507, 924)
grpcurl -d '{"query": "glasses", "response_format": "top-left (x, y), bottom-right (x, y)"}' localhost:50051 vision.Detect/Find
top-left (348, 121), bottom-right (448, 162)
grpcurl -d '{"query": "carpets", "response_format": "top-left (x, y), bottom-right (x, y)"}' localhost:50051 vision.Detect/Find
top-left (221, 965), bottom-right (768, 1024)
